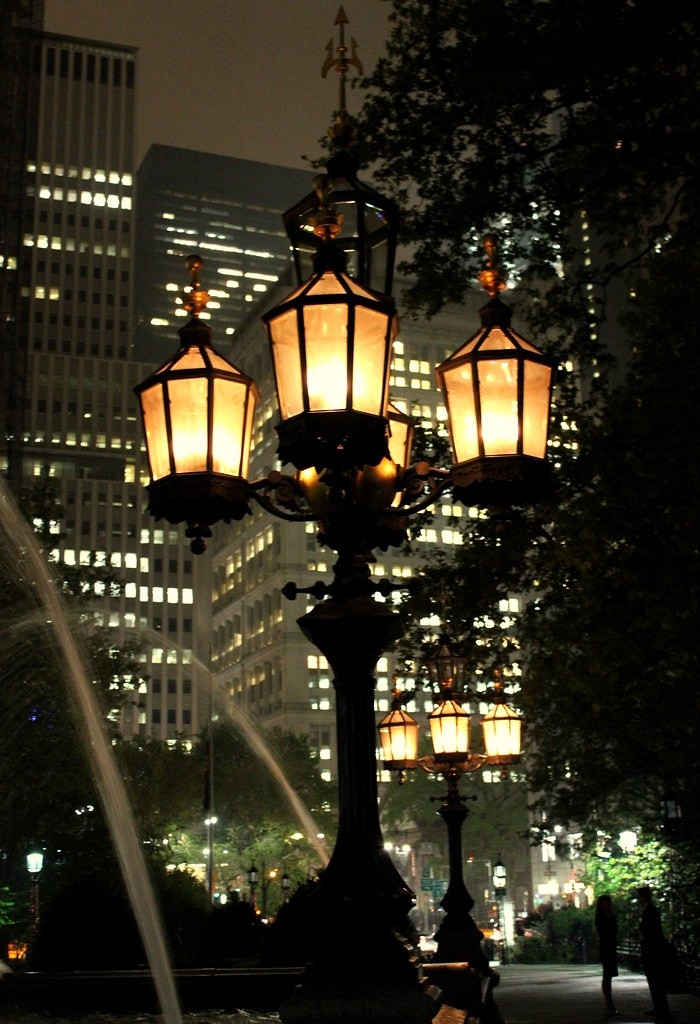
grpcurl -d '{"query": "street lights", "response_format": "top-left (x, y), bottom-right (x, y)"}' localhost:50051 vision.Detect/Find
top-left (619, 828), bottom-right (636, 971)
top-left (246, 858), bottom-right (260, 911)
top-left (132, 5), bottom-right (563, 1024)
top-left (205, 817), bottom-right (218, 903)
top-left (376, 578), bottom-right (526, 987)
top-left (492, 852), bottom-right (507, 965)
top-left (25, 848), bottom-right (44, 931)
top-left (281, 873), bottom-right (291, 905)
top-left (291, 833), bottom-right (325, 878)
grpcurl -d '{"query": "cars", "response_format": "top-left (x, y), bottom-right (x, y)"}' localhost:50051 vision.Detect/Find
top-left (477, 922), bottom-right (501, 940)
top-left (417, 933), bottom-right (438, 953)
top-left (493, 929), bottom-right (545, 953)
top-left (411, 905), bottom-right (443, 926)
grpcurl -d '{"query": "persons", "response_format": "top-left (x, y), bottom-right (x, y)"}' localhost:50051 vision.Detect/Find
top-left (592, 894), bottom-right (626, 1016)
top-left (637, 888), bottom-right (678, 1015)
